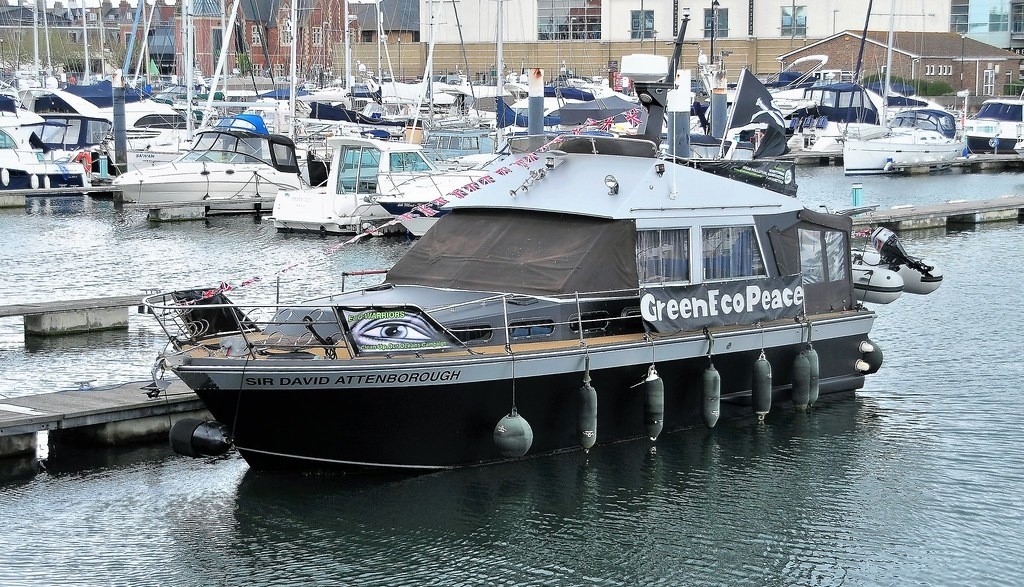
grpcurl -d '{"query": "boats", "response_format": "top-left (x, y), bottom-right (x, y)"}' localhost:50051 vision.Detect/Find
top-left (139, 133), bottom-right (878, 489)
top-left (801, 242), bottom-right (941, 301)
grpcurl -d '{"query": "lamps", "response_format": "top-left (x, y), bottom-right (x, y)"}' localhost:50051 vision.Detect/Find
top-left (605, 175), bottom-right (618, 195)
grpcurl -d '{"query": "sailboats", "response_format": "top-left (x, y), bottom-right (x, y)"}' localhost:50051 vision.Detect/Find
top-left (0, 0), bottom-right (1024, 234)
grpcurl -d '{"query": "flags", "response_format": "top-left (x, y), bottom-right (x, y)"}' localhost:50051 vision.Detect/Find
top-left (190, 110), bottom-right (644, 304)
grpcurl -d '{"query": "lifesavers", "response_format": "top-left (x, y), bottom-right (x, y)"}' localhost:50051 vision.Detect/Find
top-left (76, 152), bottom-right (92, 172)
